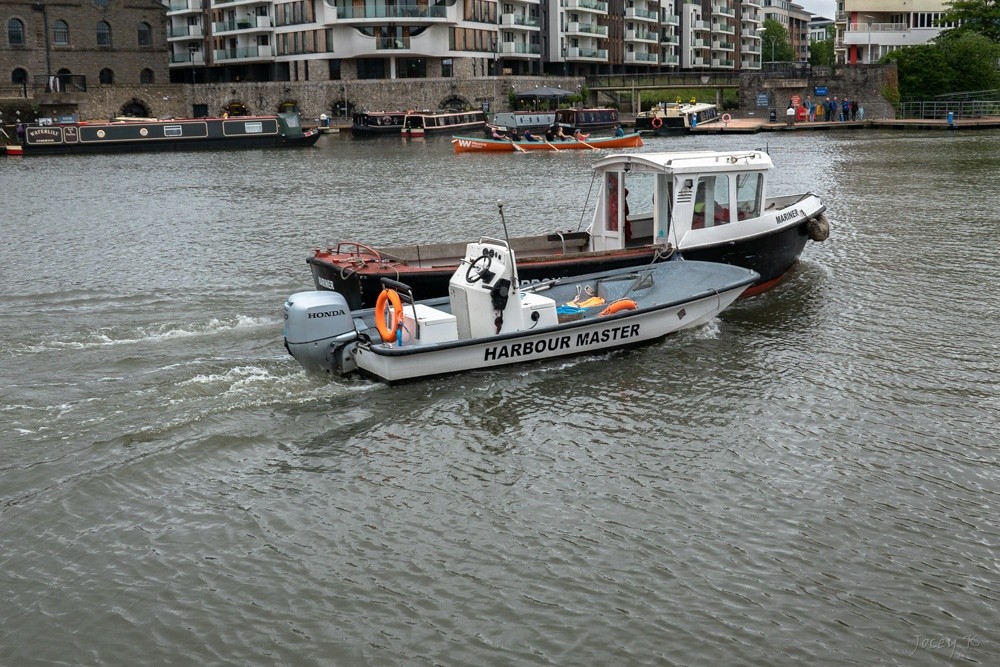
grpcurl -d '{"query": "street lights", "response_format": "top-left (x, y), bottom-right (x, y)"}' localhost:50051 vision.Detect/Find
top-left (189, 46), bottom-right (197, 84)
top-left (863, 14), bottom-right (874, 64)
top-left (562, 42), bottom-right (567, 77)
top-left (490, 37), bottom-right (499, 76)
top-left (768, 36), bottom-right (778, 72)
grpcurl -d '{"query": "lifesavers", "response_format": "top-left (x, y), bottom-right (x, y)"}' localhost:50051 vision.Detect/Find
top-left (375, 288), bottom-right (404, 343)
top-left (652, 118), bottom-right (662, 128)
top-left (78, 122), bottom-right (87, 124)
top-left (598, 299), bottom-right (637, 316)
top-left (722, 113), bottom-right (731, 123)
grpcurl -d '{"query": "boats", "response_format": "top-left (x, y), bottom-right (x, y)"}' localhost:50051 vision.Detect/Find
top-left (284, 199), bottom-right (762, 386)
top-left (305, 150), bottom-right (830, 314)
top-left (3, 145), bottom-right (25, 155)
top-left (451, 129), bottom-right (643, 153)
top-left (634, 96), bottom-right (720, 136)
top-left (20, 112), bottom-right (321, 156)
top-left (549, 108), bottom-right (622, 135)
top-left (493, 111), bottom-right (562, 136)
top-left (401, 110), bottom-right (486, 137)
top-left (350, 111), bottom-right (410, 138)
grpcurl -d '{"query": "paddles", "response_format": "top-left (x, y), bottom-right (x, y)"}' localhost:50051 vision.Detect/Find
top-left (505, 136), bottom-right (525, 152)
top-left (539, 137), bottom-right (558, 151)
top-left (571, 136), bottom-right (601, 151)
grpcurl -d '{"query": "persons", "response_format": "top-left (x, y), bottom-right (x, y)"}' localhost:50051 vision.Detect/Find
top-left (788, 96), bottom-right (858, 122)
top-left (557, 126), bottom-right (572, 140)
top-left (613, 124), bottom-right (624, 137)
top-left (491, 127), bottom-right (505, 140)
top-left (546, 129), bottom-right (557, 141)
top-left (524, 129), bottom-right (542, 141)
top-left (574, 129), bottom-right (590, 141)
top-left (512, 129), bottom-right (524, 141)
